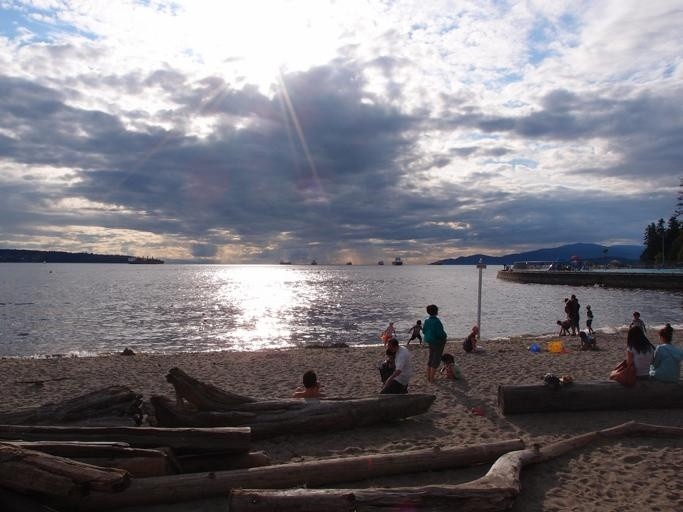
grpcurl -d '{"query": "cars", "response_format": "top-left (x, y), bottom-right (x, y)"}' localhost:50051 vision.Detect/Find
top-left (549, 262), bottom-right (593, 271)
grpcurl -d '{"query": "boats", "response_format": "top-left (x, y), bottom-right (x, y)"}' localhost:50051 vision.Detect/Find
top-left (280, 259), bottom-right (291, 264)
top-left (311, 259), bottom-right (317, 265)
top-left (131, 256), bottom-right (163, 264)
top-left (346, 261), bottom-right (352, 265)
top-left (379, 260), bottom-right (383, 264)
top-left (392, 256), bottom-right (403, 265)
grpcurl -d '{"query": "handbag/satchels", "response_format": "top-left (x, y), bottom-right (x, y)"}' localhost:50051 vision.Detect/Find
top-left (609, 360), bottom-right (636, 385)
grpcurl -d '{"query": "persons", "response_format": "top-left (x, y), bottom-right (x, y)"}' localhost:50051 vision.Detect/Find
top-left (586, 303), bottom-right (596, 335)
top-left (624, 326), bottom-right (656, 379)
top-left (567, 295), bottom-right (576, 332)
top-left (628, 311), bottom-right (647, 334)
top-left (406, 320), bottom-right (425, 345)
top-left (422, 304), bottom-right (448, 384)
top-left (564, 297), bottom-right (573, 318)
top-left (292, 368), bottom-right (326, 400)
top-left (377, 338), bottom-right (410, 394)
top-left (380, 321), bottom-right (398, 349)
top-left (648, 328), bottom-right (683, 383)
top-left (569, 299), bottom-right (580, 337)
top-left (439, 353), bottom-right (462, 382)
top-left (463, 325), bottom-right (480, 354)
top-left (557, 319), bottom-right (571, 337)
top-left (579, 331), bottom-right (596, 351)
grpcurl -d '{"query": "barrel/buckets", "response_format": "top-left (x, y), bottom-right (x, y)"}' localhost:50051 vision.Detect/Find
top-left (546, 341), bottom-right (562, 352)
